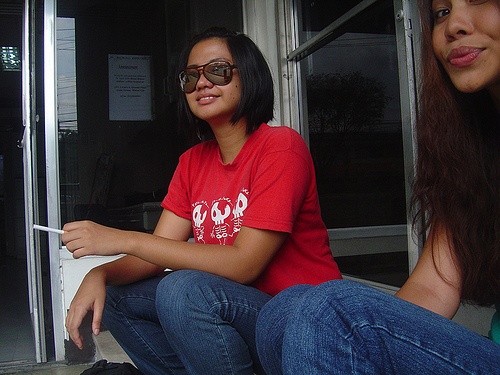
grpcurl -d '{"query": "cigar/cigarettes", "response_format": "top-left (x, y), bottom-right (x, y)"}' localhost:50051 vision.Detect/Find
top-left (33, 224), bottom-right (68, 235)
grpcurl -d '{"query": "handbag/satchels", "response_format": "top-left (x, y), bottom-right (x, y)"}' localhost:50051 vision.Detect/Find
top-left (80, 360), bottom-right (144, 375)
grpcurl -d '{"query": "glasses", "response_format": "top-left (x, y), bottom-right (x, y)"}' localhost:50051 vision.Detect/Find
top-left (175, 61), bottom-right (238, 93)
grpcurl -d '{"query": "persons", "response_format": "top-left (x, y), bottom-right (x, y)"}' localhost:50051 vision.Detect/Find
top-left (256, 0), bottom-right (500, 375)
top-left (61, 29), bottom-right (343, 375)
